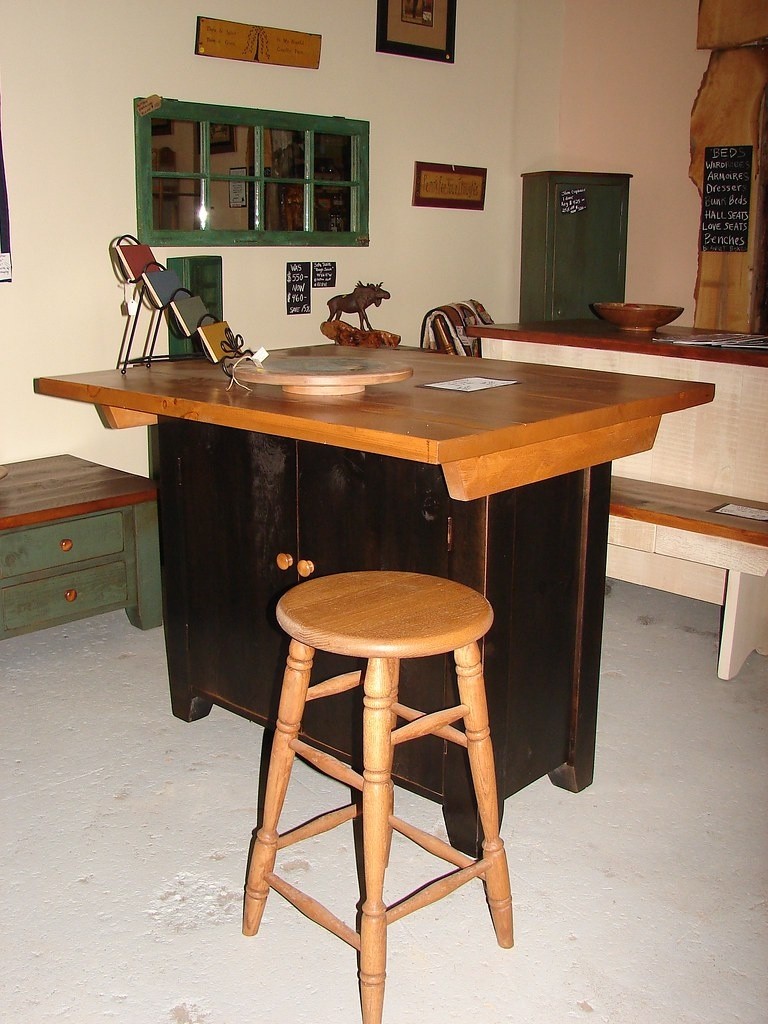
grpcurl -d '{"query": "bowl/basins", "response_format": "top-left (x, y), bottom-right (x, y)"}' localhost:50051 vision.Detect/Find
top-left (589, 303), bottom-right (684, 333)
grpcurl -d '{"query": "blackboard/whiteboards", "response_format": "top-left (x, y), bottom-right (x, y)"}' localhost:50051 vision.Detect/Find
top-left (561, 188), bottom-right (586, 214)
top-left (286, 262), bottom-right (311, 315)
top-left (312, 261), bottom-right (336, 288)
top-left (701, 145), bottom-right (753, 252)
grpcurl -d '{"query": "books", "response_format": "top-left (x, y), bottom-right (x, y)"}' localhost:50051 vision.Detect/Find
top-left (142, 270), bottom-right (187, 308)
top-left (170, 295), bottom-right (213, 337)
top-left (115, 244), bottom-right (161, 281)
top-left (196, 321), bottom-right (243, 364)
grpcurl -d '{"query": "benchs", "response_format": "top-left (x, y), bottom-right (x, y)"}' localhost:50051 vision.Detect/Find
top-left (607, 473), bottom-right (768, 681)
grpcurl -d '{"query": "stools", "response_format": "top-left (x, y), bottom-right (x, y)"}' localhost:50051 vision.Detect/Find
top-left (240, 571), bottom-right (514, 1023)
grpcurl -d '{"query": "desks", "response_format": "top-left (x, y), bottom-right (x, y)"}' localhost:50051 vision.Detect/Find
top-left (33, 341), bottom-right (716, 861)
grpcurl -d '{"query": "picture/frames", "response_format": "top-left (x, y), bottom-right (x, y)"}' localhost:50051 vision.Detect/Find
top-left (377, 0), bottom-right (458, 65)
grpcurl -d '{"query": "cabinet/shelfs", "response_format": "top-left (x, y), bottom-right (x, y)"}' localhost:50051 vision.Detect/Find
top-left (0, 452), bottom-right (164, 647)
